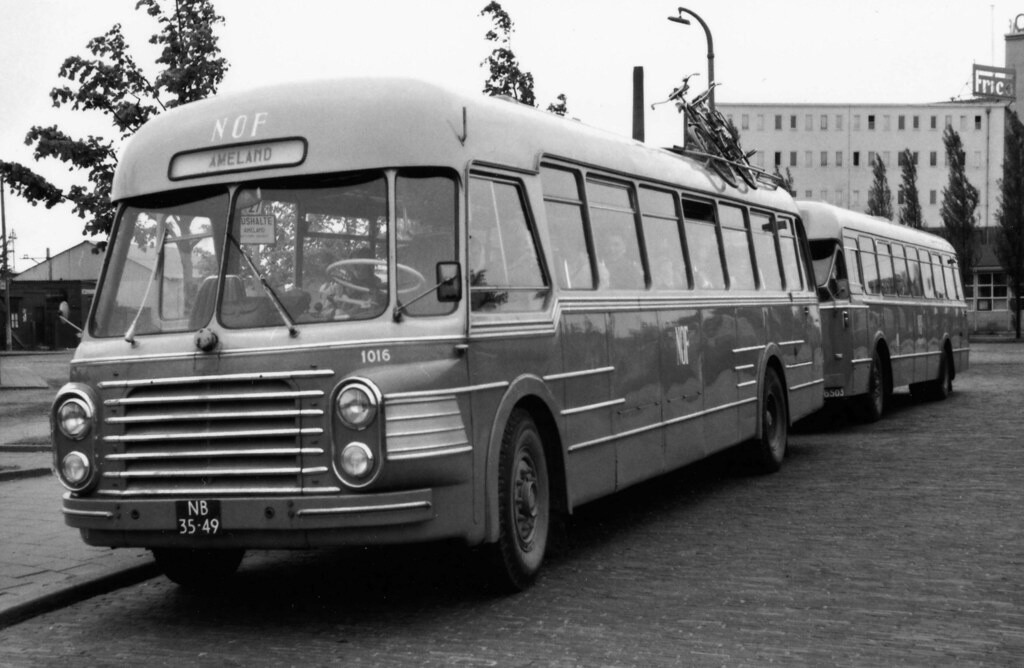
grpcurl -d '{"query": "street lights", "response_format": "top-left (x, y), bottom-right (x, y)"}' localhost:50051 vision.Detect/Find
top-left (668, 7), bottom-right (714, 113)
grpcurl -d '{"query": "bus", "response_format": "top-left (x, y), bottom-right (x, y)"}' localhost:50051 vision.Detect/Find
top-left (52, 75), bottom-right (826, 591)
top-left (796, 201), bottom-right (971, 420)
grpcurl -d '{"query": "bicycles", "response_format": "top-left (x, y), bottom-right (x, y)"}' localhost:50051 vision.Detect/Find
top-left (651, 72), bottom-right (758, 190)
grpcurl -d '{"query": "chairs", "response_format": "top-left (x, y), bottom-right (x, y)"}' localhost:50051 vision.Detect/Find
top-left (188, 275), bottom-right (246, 329)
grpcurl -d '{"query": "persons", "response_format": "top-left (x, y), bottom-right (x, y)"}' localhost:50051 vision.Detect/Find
top-left (598, 231), bottom-right (740, 289)
top-left (338, 250), bottom-right (387, 308)
